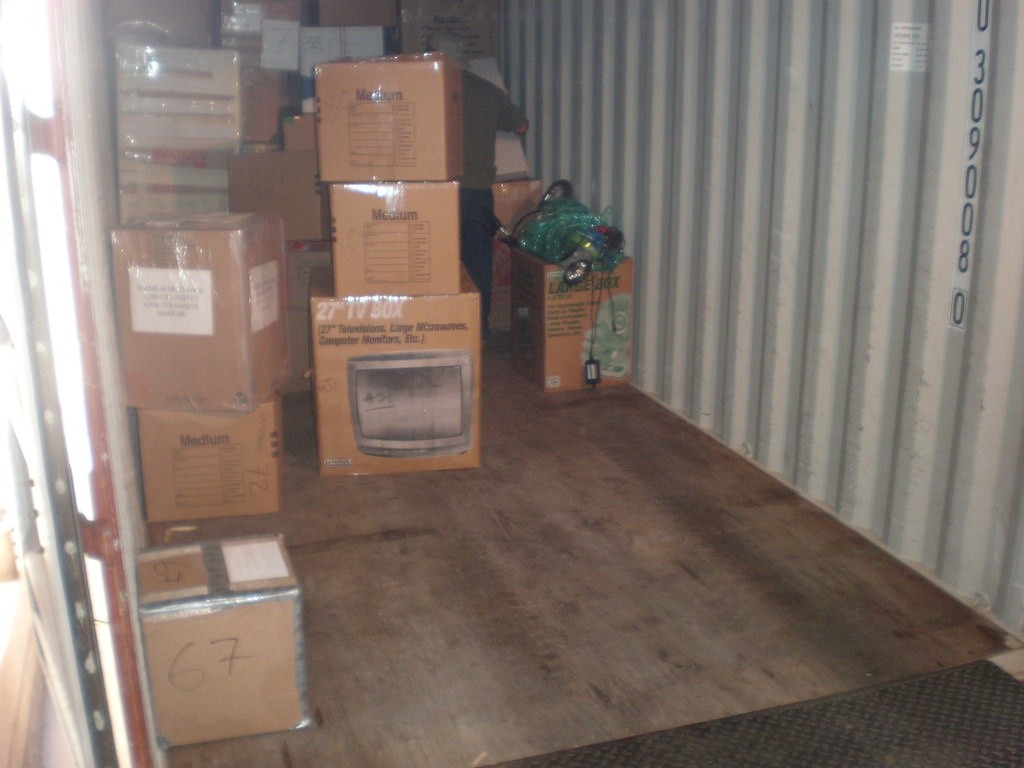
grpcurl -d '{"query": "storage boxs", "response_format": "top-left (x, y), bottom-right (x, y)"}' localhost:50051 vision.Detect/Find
top-left (328, 182), bottom-right (462, 296)
top-left (314, 51), bottom-right (463, 182)
top-left (509, 246), bottom-right (632, 393)
top-left (218, 1), bottom-right (402, 401)
top-left (109, 211), bottom-right (289, 412)
top-left (135, 532), bottom-right (309, 749)
top-left (115, 43), bottom-right (280, 148)
top-left (308, 259), bottom-right (479, 477)
top-left (459, 178), bottom-right (541, 333)
top-left (115, 145), bottom-right (283, 217)
top-left (467, 55), bottom-right (529, 177)
top-left (136, 388), bottom-right (278, 524)
top-left (103, 0), bottom-right (211, 49)
top-left (388, 1), bottom-right (501, 71)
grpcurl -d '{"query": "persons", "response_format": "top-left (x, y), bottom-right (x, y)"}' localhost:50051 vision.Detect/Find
top-left (437, 43), bottom-right (529, 328)
